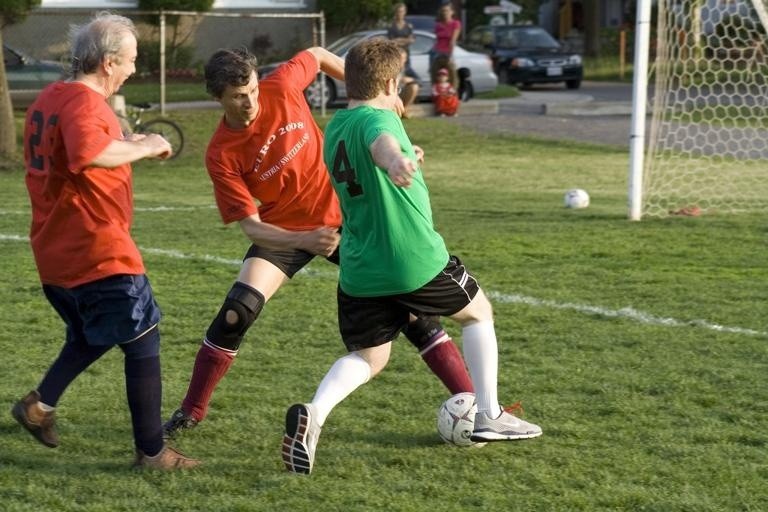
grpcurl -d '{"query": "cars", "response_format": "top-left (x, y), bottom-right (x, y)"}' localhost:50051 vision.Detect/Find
top-left (377, 16), bottom-right (438, 36)
top-left (255, 29), bottom-right (499, 110)
top-left (456, 25), bottom-right (583, 89)
top-left (2, 42), bottom-right (73, 109)
top-left (710, 29), bottom-right (762, 62)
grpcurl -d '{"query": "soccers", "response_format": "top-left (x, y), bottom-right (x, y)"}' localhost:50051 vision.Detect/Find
top-left (565, 188), bottom-right (589, 209)
top-left (437, 393), bottom-right (488, 449)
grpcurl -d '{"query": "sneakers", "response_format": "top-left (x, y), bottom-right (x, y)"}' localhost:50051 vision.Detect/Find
top-left (470, 406), bottom-right (543, 442)
top-left (161, 408), bottom-right (200, 437)
top-left (282, 404), bottom-right (321, 475)
top-left (136, 445), bottom-right (200, 468)
top-left (12, 390), bottom-right (59, 448)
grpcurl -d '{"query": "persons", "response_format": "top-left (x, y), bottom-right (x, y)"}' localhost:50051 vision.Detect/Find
top-left (386, 1), bottom-right (419, 89)
top-left (12, 11), bottom-right (198, 474)
top-left (393, 47), bottom-right (424, 119)
top-left (427, 2), bottom-right (462, 84)
top-left (431, 67), bottom-right (460, 118)
top-left (157, 45), bottom-right (479, 446)
top-left (283, 36), bottom-right (546, 476)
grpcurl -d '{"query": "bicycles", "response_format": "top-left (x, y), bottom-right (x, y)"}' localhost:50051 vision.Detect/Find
top-left (114, 102), bottom-right (184, 161)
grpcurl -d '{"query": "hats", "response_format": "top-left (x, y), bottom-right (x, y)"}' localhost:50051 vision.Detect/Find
top-left (436, 67), bottom-right (449, 76)
